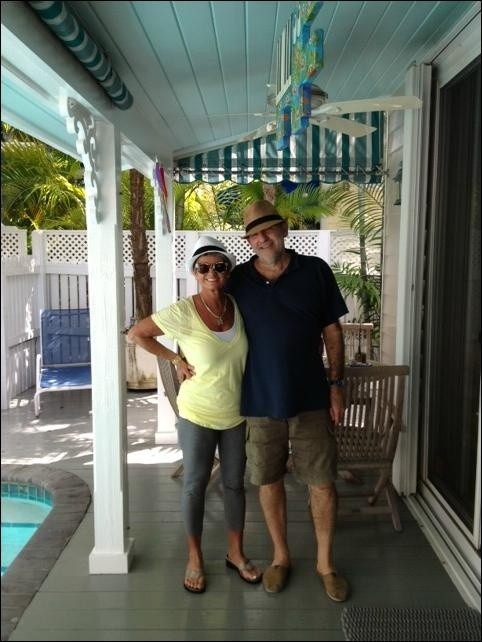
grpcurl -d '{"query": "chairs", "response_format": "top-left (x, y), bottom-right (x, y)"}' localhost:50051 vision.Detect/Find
top-left (34, 308), bottom-right (93, 419)
top-left (306, 320), bottom-right (412, 534)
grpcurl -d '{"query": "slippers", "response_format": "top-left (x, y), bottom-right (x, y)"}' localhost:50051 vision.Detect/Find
top-left (263, 565), bottom-right (287, 592)
top-left (226, 553), bottom-right (262, 584)
top-left (184, 568), bottom-right (206, 594)
top-left (319, 570), bottom-right (349, 601)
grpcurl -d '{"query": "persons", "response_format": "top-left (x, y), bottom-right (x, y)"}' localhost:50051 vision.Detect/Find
top-left (128, 235), bottom-right (263, 594)
top-left (224, 200), bottom-right (351, 602)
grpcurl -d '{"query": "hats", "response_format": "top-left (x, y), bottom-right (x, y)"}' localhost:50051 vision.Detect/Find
top-left (189, 235), bottom-right (236, 280)
top-left (239, 200), bottom-right (288, 238)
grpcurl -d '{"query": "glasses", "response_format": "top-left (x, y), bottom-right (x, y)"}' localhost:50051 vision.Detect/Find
top-left (195, 262), bottom-right (228, 274)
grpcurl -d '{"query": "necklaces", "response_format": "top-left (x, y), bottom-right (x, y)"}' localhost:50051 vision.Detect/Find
top-left (200, 294), bottom-right (227, 326)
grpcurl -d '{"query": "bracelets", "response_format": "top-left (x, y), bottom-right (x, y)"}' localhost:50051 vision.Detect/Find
top-left (172, 354), bottom-right (182, 365)
top-left (328, 378), bottom-right (345, 387)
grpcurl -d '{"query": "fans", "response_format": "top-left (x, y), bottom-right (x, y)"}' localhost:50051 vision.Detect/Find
top-left (241, 93), bottom-right (424, 142)
